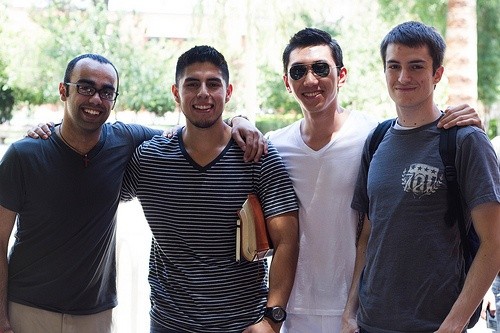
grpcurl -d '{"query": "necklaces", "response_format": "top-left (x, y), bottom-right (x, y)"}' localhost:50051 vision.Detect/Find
top-left (59, 122), bottom-right (88, 157)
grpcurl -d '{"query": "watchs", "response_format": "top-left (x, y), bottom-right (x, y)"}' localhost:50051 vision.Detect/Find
top-left (264, 306), bottom-right (287, 323)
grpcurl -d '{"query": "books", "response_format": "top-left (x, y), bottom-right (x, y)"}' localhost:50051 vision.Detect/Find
top-left (237, 194), bottom-right (273, 262)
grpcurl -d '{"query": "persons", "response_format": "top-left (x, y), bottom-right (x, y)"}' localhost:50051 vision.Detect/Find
top-left (0, 54), bottom-right (268, 333)
top-left (342, 21), bottom-right (500, 333)
top-left (160, 28), bottom-right (486, 333)
top-left (24, 44), bottom-right (299, 333)
top-left (480, 134), bottom-right (500, 333)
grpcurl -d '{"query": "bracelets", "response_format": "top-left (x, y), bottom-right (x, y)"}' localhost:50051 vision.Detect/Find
top-left (228, 114), bottom-right (250, 127)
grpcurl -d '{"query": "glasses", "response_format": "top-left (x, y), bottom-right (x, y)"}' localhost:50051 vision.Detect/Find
top-left (64, 82), bottom-right (119, 102)
top-left (285, 62), bottom-right (341, 82)
